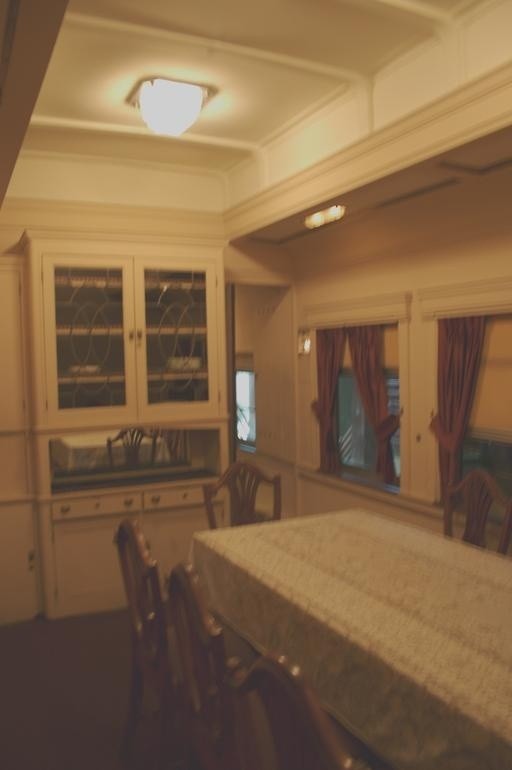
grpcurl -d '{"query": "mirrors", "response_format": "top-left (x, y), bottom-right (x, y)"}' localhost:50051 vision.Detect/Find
top-left (41, 424), bottom-right (227, 492)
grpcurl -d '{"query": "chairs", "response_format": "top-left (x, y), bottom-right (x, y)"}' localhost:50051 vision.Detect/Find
top-left (238, 647), bottom-right (362, 769)
top-left (111, 514), bottom-right (216, 770)
top-left (440, 466), bottom-right (512, 556)
top-left (195, 462), bottom-right (288, 526)
top-left (163, 557), bottom-right (278, 769)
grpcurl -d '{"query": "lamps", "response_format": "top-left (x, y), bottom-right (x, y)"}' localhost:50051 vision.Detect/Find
top-left (128, 77), bottom-right (219, 140)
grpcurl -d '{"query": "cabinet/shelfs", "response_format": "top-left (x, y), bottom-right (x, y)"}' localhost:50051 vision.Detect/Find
top-left (4, 232), bottom-right (232, 427)
top-left (46, 475), bottom-right (227, 615)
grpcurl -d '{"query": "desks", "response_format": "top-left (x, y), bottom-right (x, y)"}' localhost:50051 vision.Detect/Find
top-left (179, 507), bottom-right (512, 770)
